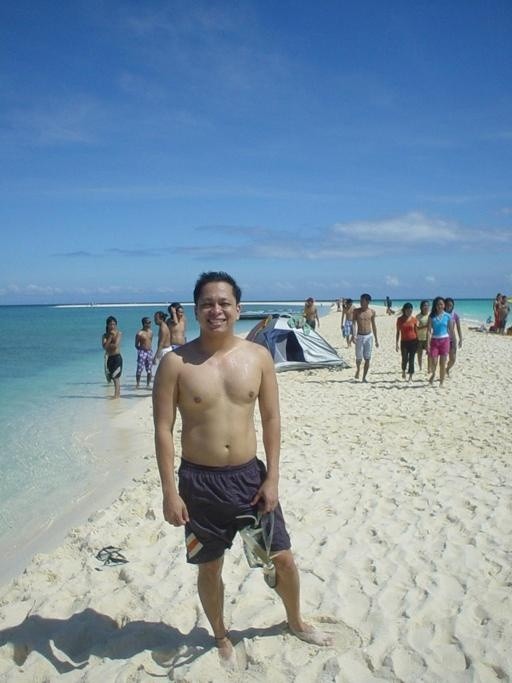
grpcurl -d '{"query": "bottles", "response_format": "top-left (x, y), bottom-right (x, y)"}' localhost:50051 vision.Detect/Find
top-left (241, 526), bottom-right (277, 587)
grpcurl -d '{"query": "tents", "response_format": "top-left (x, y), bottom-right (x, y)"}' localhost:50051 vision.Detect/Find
top-left (243, 311), bottom-right (352, 375)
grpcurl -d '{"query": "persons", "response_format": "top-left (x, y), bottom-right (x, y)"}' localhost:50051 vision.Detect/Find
top-left (135, 302), bottom-right (186, 389)
top-left (386, 297), bottom-right (394, 315)
top-left (351, 294), bottom-right (379, 383)
top-left (101, 315), bottom-right (123, 399)
top-left (152, 272), bottom-right (333, 672)
top-left (336, 297), bottom-right (350, 310)
top-left (396, 296), bottom-right (463, 387)
top-left (496, 296), bottom-right (510, 335)
top-left (339, 299), bottom-right (357, 348)
top-left (493, 293), bottom-right (502, 334)
top-left (300, 297), bottom-right (319, 331)
